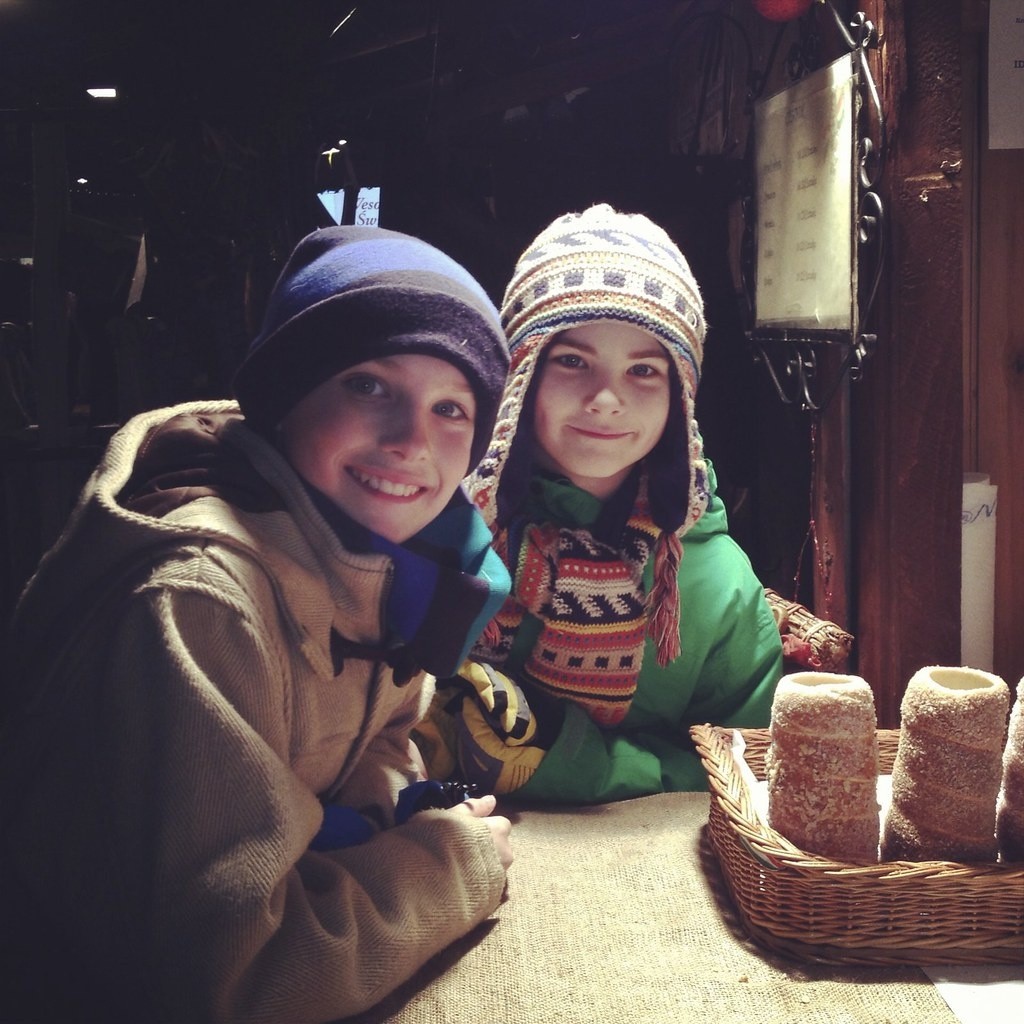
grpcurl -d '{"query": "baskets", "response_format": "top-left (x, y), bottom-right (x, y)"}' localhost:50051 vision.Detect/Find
top-left (688, 724), bottom-right (1024, 964)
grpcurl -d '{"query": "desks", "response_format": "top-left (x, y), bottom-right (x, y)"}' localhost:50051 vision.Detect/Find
top-left (320, 790), bottom-right (955, 1024)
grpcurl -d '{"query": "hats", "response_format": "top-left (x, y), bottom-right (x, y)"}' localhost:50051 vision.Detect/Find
top-left (234, 226), bottom-right (511, 479)
top-left (457, 204), bottom-right (710, 667)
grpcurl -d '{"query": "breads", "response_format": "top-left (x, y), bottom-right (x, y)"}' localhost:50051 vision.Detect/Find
top-left (761, 662), bottom-right (1024, 865)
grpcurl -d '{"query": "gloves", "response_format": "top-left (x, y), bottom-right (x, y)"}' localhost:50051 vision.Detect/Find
top-left (457, 695), bottom-right (548, 796)
top-left (456, 658), bottom-right (538, 747)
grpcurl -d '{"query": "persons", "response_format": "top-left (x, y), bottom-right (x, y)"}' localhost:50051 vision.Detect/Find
top-left (0, 226), bottom-right (514, 1024)
top-left (458, 203), bottom-right (786, 808)
